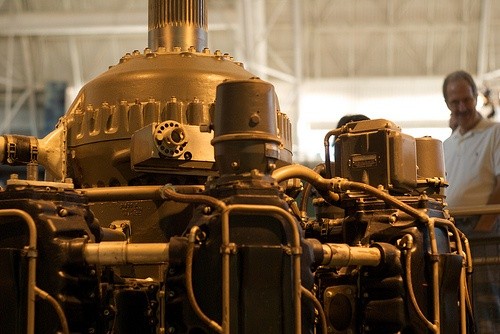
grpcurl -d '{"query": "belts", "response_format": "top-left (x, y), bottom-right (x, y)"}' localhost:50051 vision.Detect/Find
top-left (454, 215), bottom-right (479, 224)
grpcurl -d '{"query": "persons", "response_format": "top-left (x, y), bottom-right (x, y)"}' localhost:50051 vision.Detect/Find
top-left (440, 70), bottom-right (500, 334)
